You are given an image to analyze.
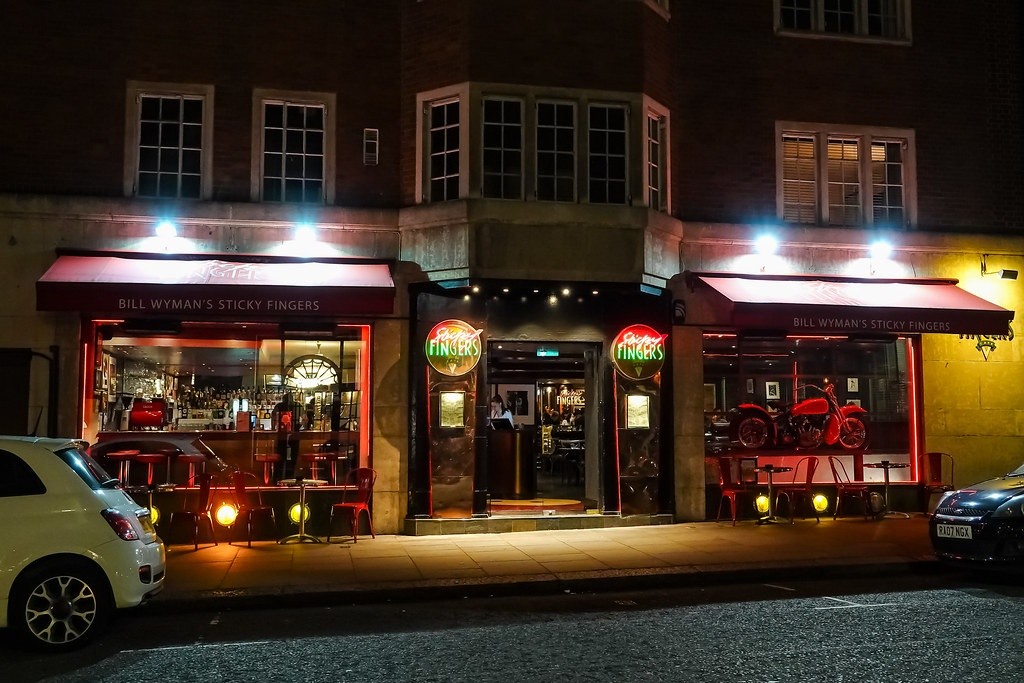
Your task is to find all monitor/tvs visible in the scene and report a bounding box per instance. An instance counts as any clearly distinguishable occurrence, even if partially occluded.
[490,418,513,430]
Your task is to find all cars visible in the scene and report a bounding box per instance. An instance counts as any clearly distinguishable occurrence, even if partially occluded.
[929,460,1024,574]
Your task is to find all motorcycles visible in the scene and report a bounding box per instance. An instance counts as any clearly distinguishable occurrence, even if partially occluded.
[728,377,874,451]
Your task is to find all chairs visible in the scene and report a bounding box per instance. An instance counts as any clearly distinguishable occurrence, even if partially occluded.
[166,473,221,550]
[714,456,763,526]
[917,453,955,515]
[828,456,874,520]
[775,457,820,525]
[225,471,278,548]
[327,468,378,544]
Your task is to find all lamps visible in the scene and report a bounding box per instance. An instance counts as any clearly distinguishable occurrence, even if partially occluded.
[980,255,1018,280]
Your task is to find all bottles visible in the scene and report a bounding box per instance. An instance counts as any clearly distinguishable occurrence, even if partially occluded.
[237,389,241,400]
[579,425,582,431]
[231,390,236,400]
[215,389,221,400]
[257,386,261,400]
[226,389,232,400]
[183,405,187,418]
[247,387,251,400]
[573,425,576,431]
[261,387,287,401]
[252,387,256,401]
[221,390,226,400]
[223,400,229,409]
[242,388,246,399]
[178,405,182,418]
[188,406,192,419]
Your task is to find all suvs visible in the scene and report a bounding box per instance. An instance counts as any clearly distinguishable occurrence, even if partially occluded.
[0,433,172,654]
[86,436,233,486]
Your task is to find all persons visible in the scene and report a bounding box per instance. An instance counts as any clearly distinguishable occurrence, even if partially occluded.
[542,406,585,426]
[271,393,310,484]
[491,394,514,428]
[306,398,326,429]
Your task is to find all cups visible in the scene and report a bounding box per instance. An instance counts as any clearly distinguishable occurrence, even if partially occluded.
[258,405,261,409]
[252,405,256,409]
[248,405,251,409]
[272,405,275,409]
[263,404,266,410]
[267,405,271,409]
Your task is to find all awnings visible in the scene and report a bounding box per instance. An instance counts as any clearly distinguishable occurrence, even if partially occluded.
[35,252,397,314]
[683,269,1015,338]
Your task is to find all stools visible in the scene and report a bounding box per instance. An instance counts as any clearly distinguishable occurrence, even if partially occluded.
[303,453,343,487]
[108,449,206,490]
[254,454,280,486]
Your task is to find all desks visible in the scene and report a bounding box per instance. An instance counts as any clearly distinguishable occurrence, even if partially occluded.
[128,484,174,517]
[861,461,911,518]
[746,464,793,524]
[278,478,328,544]
